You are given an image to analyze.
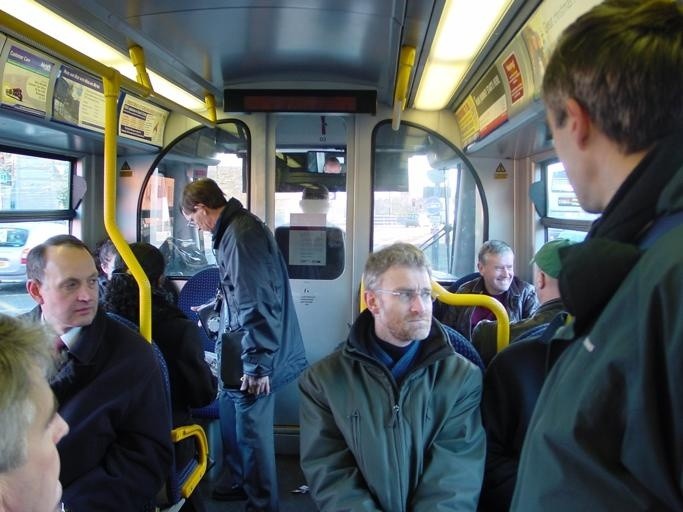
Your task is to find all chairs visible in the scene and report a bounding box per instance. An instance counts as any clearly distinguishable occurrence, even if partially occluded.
[430,312,494,511]
[175,267,251,481]
[98,311,209,511]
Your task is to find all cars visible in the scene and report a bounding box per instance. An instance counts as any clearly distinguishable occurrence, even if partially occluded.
[548,229,586,242]
[0,220,69,290]
[405,212,419,227]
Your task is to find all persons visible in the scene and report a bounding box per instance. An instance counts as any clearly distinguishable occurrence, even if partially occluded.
[295,240,489,512]
[177,177,311,512]
[507,1,683,510]
[471,238,579,360]
[299,181,330,219]
[0,313,72,512]
[9,233,175,512]
[442,238,539,344]
[97,241,219,472]
[89,236,128,305]
[323,157,341,174]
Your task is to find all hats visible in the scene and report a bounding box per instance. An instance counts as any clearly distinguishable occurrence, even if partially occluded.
[529,238,578,278]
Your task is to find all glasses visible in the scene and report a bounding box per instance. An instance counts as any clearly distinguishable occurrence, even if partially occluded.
[378,287,438,305]
[187,213,197,229]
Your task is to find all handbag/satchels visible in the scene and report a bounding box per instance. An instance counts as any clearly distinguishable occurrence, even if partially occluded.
[220,332,247,388]
